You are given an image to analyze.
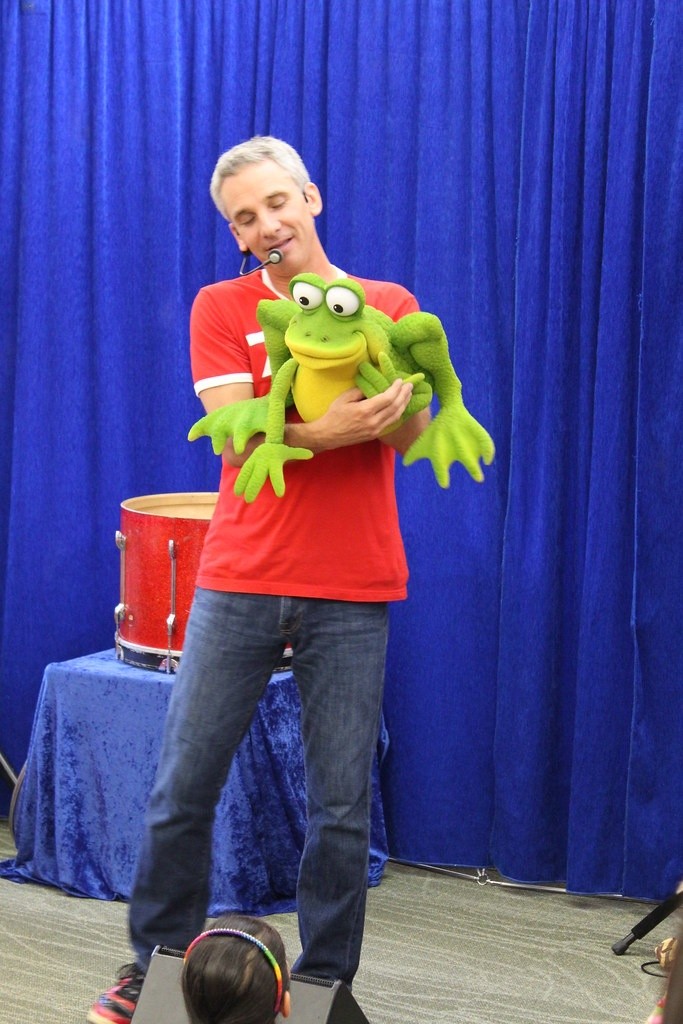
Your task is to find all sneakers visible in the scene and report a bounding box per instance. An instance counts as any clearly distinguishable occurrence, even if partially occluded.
[86,963,145,1024]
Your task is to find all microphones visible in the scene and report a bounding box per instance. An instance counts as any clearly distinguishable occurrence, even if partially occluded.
[239,248,284,275]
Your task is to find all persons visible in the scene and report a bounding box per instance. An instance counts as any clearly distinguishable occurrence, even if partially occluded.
[87,136,432,1024]
[180,914,291,1024]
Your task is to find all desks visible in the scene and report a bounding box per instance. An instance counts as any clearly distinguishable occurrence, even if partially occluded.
[0,648,390,918]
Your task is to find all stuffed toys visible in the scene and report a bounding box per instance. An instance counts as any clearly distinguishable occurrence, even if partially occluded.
[187,273,494,489]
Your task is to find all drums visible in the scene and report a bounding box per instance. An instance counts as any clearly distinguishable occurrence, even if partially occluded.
[111,487,297,676]
